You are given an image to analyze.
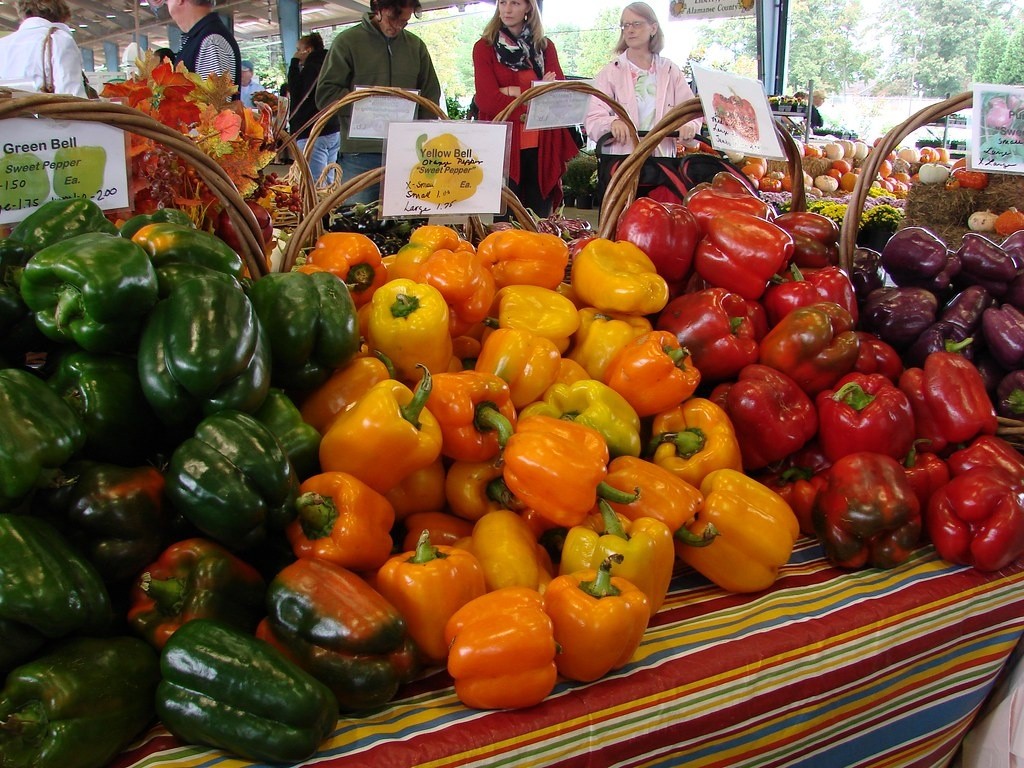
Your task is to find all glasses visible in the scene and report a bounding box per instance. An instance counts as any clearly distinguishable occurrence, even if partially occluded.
[619,20,648,30]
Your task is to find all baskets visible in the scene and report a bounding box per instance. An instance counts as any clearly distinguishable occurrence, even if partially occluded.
[0,81,1024,457]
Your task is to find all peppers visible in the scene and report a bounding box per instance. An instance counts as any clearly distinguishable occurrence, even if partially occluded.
[0,171,1024,768]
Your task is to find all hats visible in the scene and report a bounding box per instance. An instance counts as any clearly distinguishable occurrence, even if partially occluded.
[241,60,254,71]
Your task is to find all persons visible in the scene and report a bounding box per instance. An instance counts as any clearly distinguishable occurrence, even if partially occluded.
[794,92,810,100]
[315,0,441,211]
[584,1,702,200]
[155,48,181,65]
[471,0,580,223]
[287,31,340,187]
[166,0,241,102]
[0,0,90,100]
[241,60,266,108]
[277,82,290,148]
[800,89,828,129]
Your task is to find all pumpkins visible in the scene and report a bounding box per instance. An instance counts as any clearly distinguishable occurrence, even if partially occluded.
[684,137,1024,234]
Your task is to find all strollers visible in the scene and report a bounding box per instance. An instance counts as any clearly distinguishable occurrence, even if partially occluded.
[594,129,762,230]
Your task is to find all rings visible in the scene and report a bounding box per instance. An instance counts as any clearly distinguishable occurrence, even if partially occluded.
[689,132,693,136]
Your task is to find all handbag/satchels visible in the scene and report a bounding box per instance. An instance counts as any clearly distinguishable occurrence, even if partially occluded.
[40,27,99,100]
[568,125,584,146]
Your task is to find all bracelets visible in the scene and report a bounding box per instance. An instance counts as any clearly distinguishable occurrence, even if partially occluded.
[507,86,510,96]
